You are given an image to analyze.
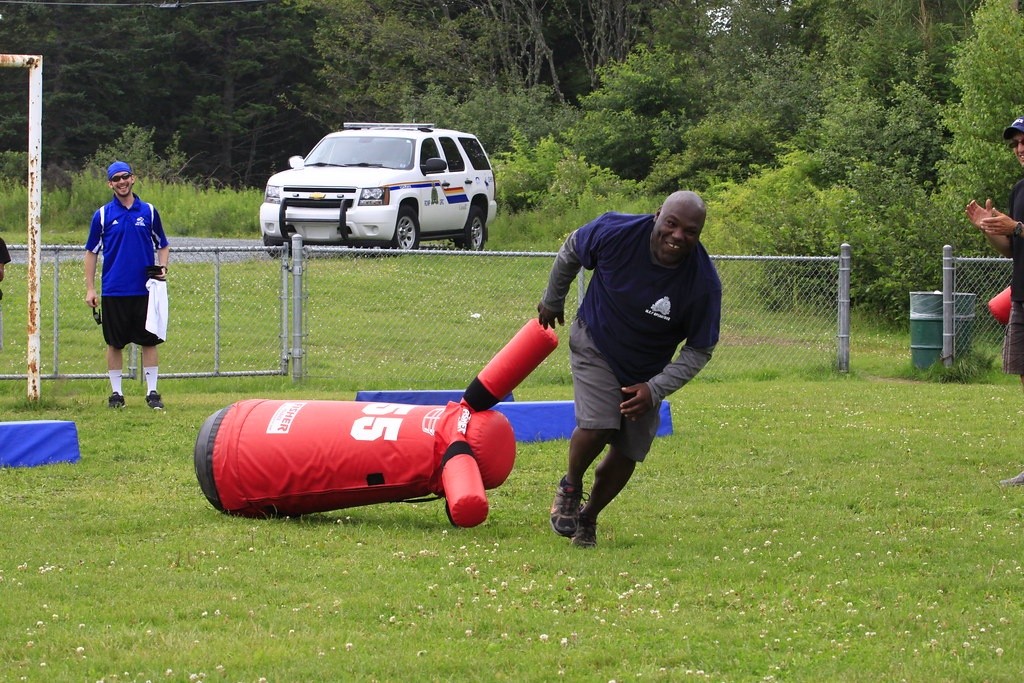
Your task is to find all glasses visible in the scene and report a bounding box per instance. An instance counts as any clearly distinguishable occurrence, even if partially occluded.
[1009,139,1024,148]
[110,173,132,182]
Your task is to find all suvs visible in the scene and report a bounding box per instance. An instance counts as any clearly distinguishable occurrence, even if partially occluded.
[258,121,498,260]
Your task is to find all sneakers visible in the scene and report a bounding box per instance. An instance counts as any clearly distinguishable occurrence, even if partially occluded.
[109,391,125,408]
[566,505,598,550]
[550,476,583,538]
[145,390,164,410]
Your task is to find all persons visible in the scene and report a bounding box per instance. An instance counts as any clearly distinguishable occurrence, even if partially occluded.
[534,191,724,549]
[83,160,171,409]
[965,117,1024,485]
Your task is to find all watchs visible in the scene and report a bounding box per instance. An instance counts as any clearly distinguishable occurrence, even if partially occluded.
[1014,220,1022,238]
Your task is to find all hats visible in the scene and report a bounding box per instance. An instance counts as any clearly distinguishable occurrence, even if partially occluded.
[1003,117,1024,139]
[108,162,132,180]
